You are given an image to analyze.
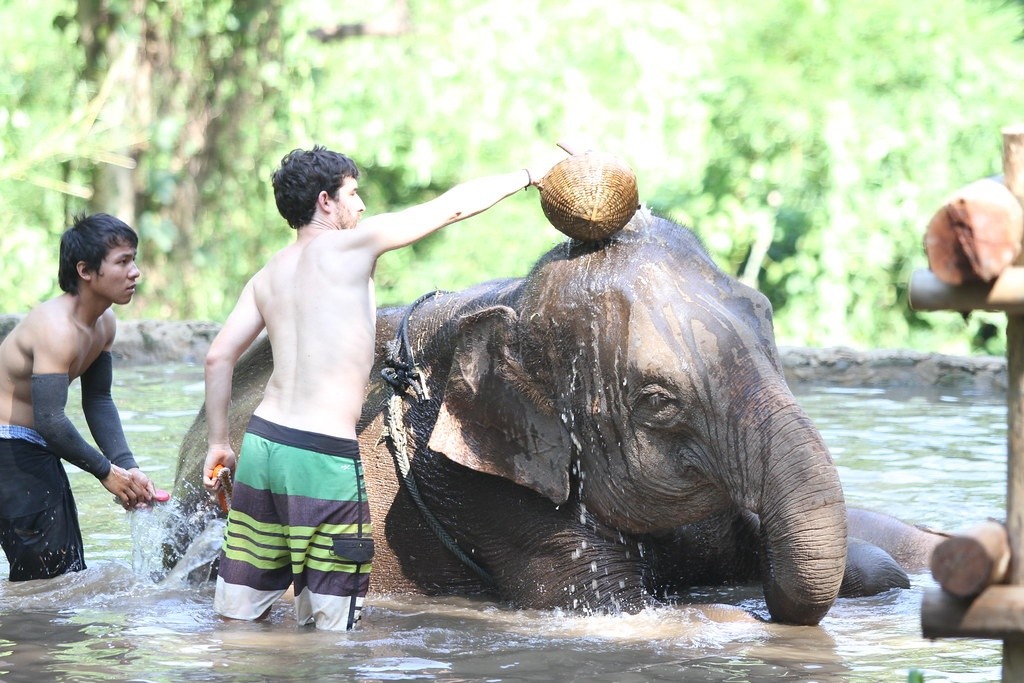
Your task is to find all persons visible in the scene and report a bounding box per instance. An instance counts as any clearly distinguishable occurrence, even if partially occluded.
[0,209,156,583]
[202,144,595,632]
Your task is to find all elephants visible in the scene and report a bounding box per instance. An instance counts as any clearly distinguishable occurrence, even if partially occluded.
[156,204,957,617]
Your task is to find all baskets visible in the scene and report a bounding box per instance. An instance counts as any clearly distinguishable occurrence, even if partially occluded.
[535,141,640,244]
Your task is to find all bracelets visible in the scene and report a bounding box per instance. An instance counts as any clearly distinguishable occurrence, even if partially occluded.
[522,169,531,191]
